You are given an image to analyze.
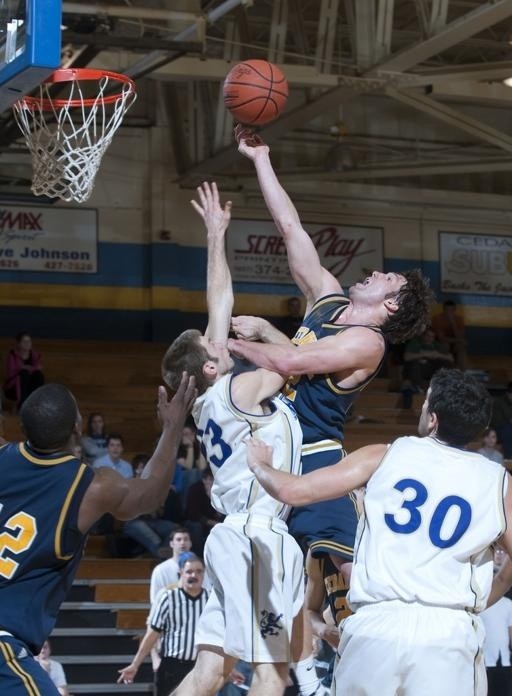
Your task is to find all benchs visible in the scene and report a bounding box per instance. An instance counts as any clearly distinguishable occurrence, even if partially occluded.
[468,350,511,395]
[48,530,161,696]
[0,333,170,466]
[345,358,434,457]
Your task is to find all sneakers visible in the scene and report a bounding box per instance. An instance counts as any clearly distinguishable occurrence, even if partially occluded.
[158,546,174,559]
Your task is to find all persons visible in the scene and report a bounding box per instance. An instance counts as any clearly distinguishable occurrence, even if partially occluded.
[164,178,307,696]
[225,120,433,696]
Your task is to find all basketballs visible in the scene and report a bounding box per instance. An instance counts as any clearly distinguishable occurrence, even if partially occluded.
[223,60,289,127]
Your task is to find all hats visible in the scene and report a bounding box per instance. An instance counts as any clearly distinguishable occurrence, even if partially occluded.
[179,551,196,568]
